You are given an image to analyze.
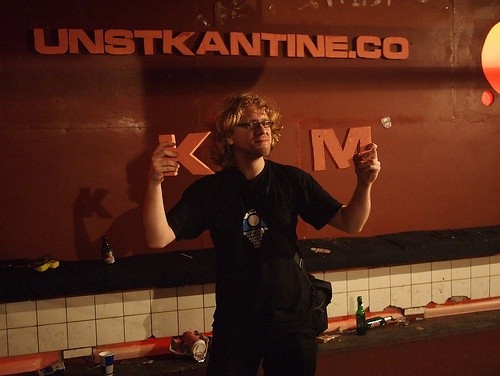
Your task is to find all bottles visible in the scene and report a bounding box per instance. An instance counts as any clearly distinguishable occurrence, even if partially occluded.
[366,316,393,329]
[101,236,115,267]
[355,296,366,335]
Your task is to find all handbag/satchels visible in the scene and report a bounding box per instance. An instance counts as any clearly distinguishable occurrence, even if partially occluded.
[308,272,333,335]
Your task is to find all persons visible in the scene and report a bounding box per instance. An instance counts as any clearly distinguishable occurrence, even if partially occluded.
[141,91,381,376]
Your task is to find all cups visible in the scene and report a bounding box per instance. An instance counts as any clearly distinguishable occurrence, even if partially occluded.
[98,351,114,376]
[182,331,206,355]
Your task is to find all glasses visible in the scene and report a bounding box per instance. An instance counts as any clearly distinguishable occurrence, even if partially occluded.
[238,121,273,130]
